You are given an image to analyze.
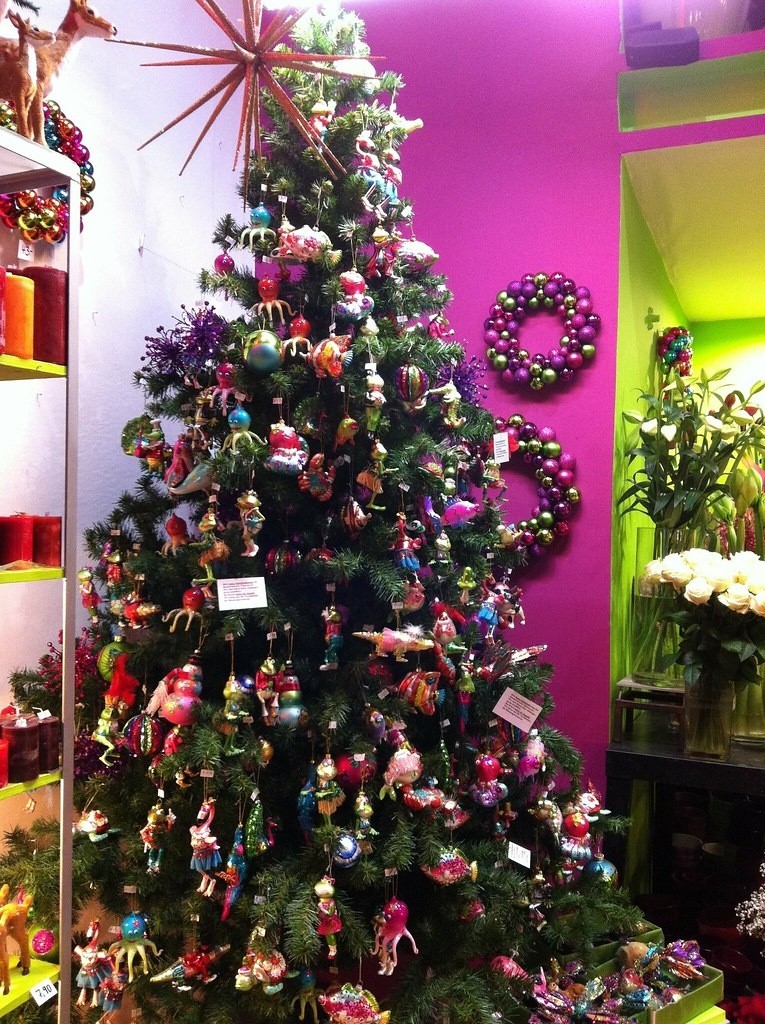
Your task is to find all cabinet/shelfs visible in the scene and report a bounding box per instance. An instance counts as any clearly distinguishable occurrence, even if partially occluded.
[0,128,87,1024]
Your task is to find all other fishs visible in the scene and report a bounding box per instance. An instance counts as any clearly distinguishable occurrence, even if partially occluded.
[379,748,424,802]
[317,984,391,1024]
[279,224,343,263]
[420,847,477,884]
[343,499,372,539]
[395,239,439,279]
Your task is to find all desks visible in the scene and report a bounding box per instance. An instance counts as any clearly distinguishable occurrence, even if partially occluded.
[602,711,765,892]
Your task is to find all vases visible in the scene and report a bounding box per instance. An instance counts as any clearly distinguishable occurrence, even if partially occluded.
[630,527,707,687]
[685,681,735,763]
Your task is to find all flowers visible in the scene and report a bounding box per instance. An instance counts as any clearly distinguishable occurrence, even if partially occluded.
[644,547,765,686]
[616,366,765,528]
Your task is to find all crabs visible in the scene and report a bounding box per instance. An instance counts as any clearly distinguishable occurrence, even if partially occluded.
[298,454,335,500]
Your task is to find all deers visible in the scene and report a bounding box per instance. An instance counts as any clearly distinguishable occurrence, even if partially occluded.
[0,0,117,147]
